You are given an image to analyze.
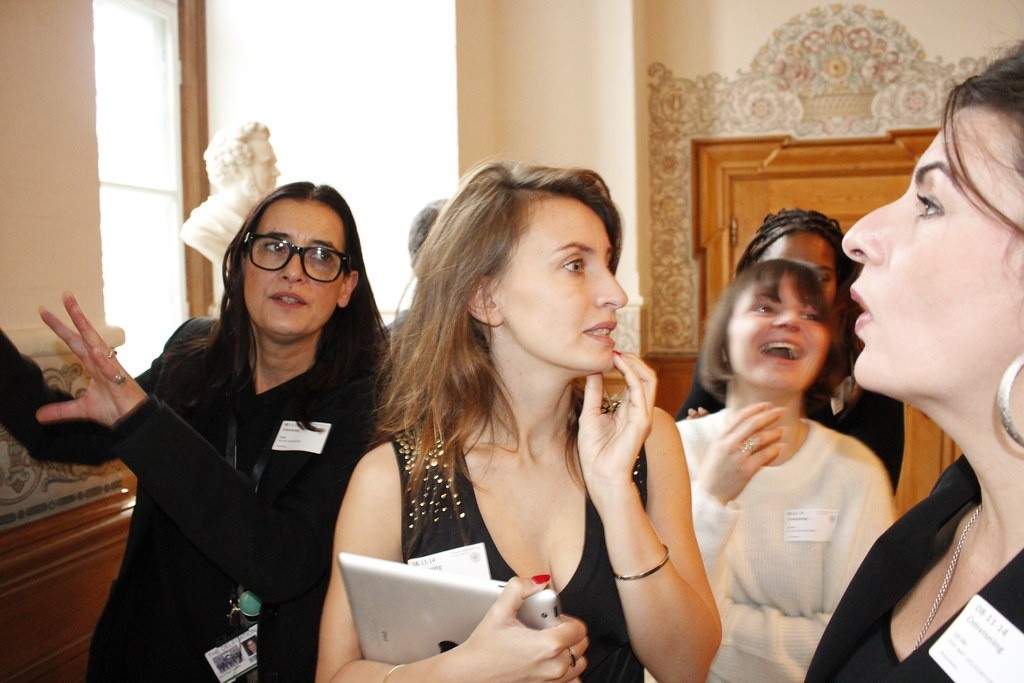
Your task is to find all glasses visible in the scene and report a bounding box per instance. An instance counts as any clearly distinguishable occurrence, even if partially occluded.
[244,232,353,283]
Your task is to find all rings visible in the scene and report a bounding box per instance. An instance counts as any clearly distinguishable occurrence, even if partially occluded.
[568,647,576,667]
[108,346,115,359]
[111,371,125,384]
[738,437,757,455]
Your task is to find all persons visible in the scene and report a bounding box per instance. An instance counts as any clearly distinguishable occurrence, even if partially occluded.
[806,42,1024,683]
[645,259,891,683]
[180,122,281,268]
[0,182,389,683]
[247,639,257,655]
[678,208,906,496]
[315,162,723,683]
[386,200,451,331]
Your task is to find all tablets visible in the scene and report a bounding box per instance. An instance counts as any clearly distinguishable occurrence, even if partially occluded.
[338,552,582,683]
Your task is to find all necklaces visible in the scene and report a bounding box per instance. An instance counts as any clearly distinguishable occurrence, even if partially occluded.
[914,504,983,651]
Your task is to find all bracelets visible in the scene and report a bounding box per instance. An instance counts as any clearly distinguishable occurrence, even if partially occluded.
[382,664,406,683]
[615,544,670,581]
